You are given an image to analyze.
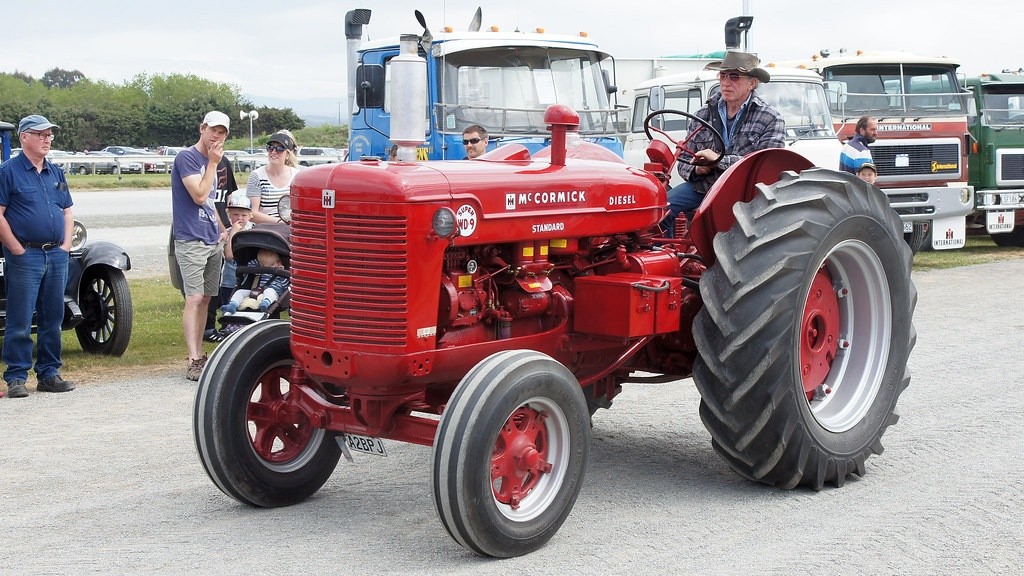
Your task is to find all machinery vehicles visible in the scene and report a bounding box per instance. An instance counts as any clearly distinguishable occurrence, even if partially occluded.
[191,100,915,560]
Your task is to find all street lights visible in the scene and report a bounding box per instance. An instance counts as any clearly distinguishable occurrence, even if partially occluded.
[240,109,259,172]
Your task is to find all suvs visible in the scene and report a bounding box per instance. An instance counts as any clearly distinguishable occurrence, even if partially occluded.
[298,147,341,167]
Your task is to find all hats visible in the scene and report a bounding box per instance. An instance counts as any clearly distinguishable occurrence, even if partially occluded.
[266,134,297,152]
[202,111,230,135]
[858,162,876,174]
[18,115,60,137]
[227,191,251,210]
[706,51,770,83]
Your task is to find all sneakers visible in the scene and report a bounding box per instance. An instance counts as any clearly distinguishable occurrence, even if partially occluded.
[199,352,207,366]
[203,329,224,342]
[186,359,202,380]
[8,379,29,397]
[37,375,75,391]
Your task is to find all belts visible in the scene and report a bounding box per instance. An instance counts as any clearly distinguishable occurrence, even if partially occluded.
[22,241,63,250]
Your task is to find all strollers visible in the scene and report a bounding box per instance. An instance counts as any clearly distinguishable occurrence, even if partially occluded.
[219,220,292,337]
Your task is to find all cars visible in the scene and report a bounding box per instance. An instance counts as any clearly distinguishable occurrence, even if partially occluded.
[10,145,187,175]
[223,149,269,172]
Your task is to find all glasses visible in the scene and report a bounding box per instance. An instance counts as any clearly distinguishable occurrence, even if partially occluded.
[265,143,288,154]
[716,72,753,82]
[462,138,483,145]
[25,132,54,140]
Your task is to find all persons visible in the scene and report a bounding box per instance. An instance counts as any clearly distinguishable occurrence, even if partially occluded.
[859,162,877,185]
[219,250,289,314]
[217,191,256,306]
[0,113,76,398]
[203,155,239,341]
[838,115,878,176]
[462,125,490,160]
[660,50,785,239]
[246,129,302,227]
[171,110,230,383]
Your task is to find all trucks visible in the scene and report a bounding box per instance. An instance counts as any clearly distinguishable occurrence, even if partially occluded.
[313,6,1024,259]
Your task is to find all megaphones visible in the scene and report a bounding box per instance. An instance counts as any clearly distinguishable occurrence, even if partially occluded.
[249,110,259,120]
[240,110,250,120]
